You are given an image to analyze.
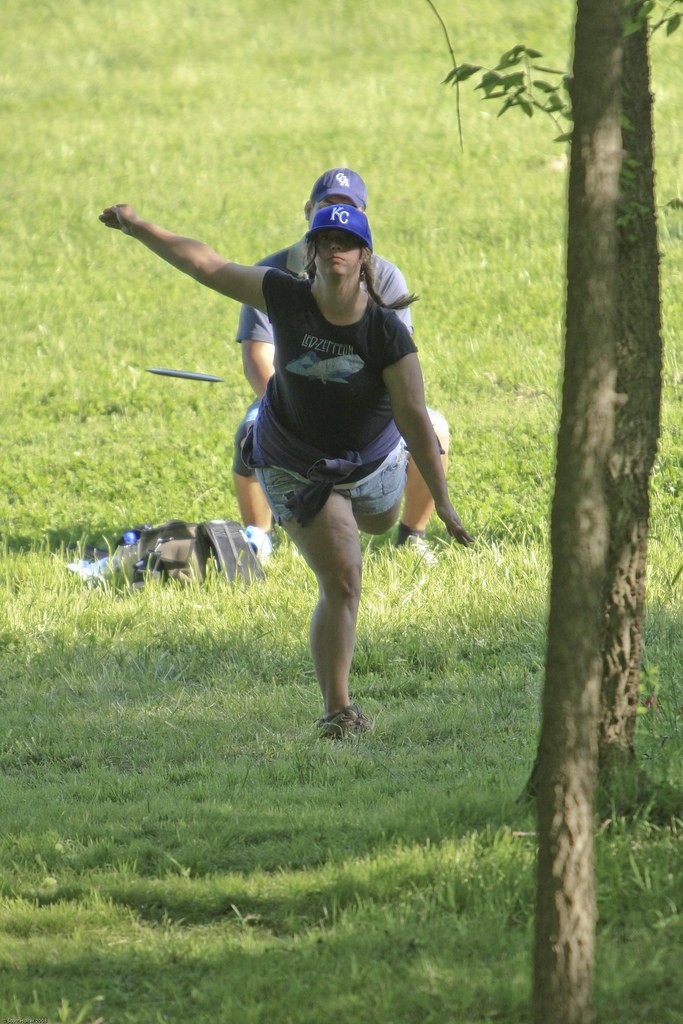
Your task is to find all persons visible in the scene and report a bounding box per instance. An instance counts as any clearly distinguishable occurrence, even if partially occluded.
[98,204,474,740]
[231,169,448,568]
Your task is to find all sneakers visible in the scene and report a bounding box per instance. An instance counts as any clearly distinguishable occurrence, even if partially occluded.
[317,704,373,741]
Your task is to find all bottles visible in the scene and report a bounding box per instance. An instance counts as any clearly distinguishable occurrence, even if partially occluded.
[120,533,138,566]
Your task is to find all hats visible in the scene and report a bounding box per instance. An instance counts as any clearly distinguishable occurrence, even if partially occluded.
[305,204,372,252]
[312,169,365,212]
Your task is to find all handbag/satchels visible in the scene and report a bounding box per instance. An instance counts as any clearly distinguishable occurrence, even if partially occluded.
[131,519,266,586]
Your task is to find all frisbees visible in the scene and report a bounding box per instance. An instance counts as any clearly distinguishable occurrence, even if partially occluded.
[145,368,224,383]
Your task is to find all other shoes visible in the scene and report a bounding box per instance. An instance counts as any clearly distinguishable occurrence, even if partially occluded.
[394,538,434,565]
